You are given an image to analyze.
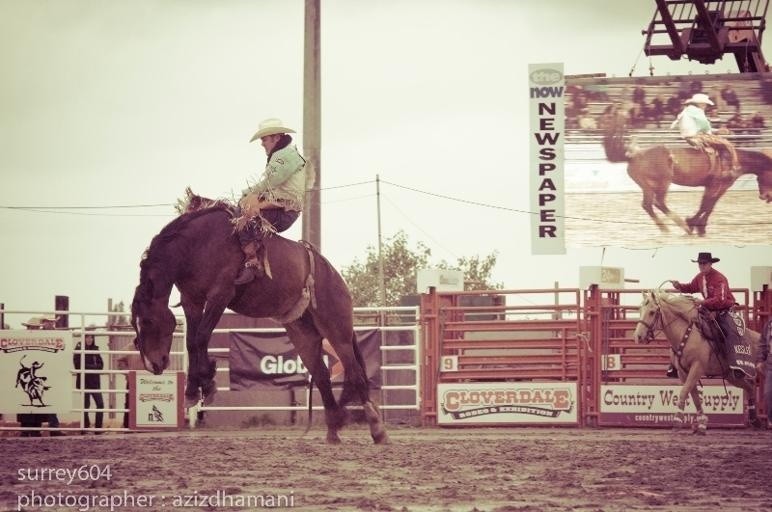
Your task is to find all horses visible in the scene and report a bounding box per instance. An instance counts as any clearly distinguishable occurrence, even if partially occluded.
[127,205,388,445]
[599,110,772,238]
[634,288,772,433]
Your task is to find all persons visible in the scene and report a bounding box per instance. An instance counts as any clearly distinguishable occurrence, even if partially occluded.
[19,316,45,437]
[40,313,65,435]
[669,251,747,382]
[229,117,310,281]
[755,319,772,425]
[117,332,146,432]
[73,334,104,433]
[563,84,771,129]
[668,92,733,178]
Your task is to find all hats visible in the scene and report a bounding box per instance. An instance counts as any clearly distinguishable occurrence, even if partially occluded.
[680,94,714,107]
[40,314,61,323]
[21,317,46,327]
[249,118,296,142]
[689,252,720,263]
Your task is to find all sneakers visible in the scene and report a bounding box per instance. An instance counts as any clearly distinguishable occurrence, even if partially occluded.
[19,428,104,437]
[666,366,677,377]
[732,366,745,379]
[233,261,267,285]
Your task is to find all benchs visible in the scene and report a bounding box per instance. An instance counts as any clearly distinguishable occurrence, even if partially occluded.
[563,77,772,149]
